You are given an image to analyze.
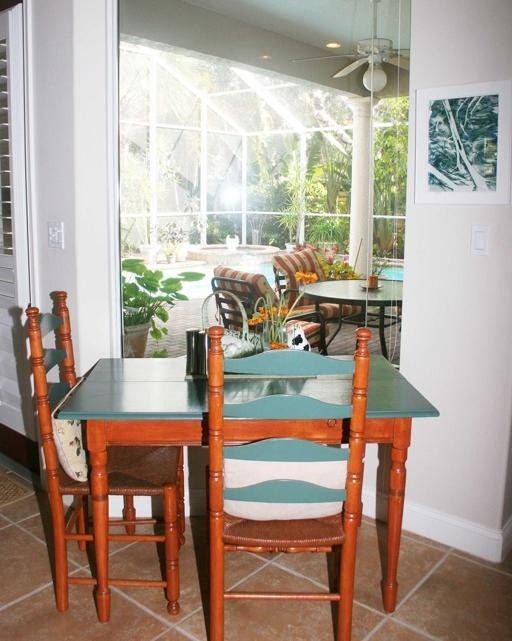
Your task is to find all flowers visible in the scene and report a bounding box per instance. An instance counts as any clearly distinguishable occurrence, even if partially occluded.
[246,269,317,350]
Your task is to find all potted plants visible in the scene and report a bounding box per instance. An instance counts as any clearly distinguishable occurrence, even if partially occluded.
[121,255,205,356]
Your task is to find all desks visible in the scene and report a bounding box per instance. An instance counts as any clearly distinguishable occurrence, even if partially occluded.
[51,355,436,620]
[297,274,405,364]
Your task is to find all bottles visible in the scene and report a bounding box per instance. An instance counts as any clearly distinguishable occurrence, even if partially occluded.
[186,328,209,376]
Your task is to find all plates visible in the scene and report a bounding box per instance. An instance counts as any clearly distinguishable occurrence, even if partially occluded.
[359,283,384,289]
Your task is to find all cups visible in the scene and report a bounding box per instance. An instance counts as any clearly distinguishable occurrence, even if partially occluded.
[367,274,378,288]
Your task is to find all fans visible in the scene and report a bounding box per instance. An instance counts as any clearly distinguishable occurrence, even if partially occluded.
[290,0,410,78]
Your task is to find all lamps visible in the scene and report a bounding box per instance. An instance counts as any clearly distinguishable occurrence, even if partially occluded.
[363,0,388,93]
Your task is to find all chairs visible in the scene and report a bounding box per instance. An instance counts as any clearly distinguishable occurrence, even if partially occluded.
[194,321,373,641]
[268,248,359,332]
[26,288,186,619]
[209,266,331,355]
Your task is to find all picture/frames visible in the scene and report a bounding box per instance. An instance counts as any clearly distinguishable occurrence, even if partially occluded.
[415,80,510,204]
